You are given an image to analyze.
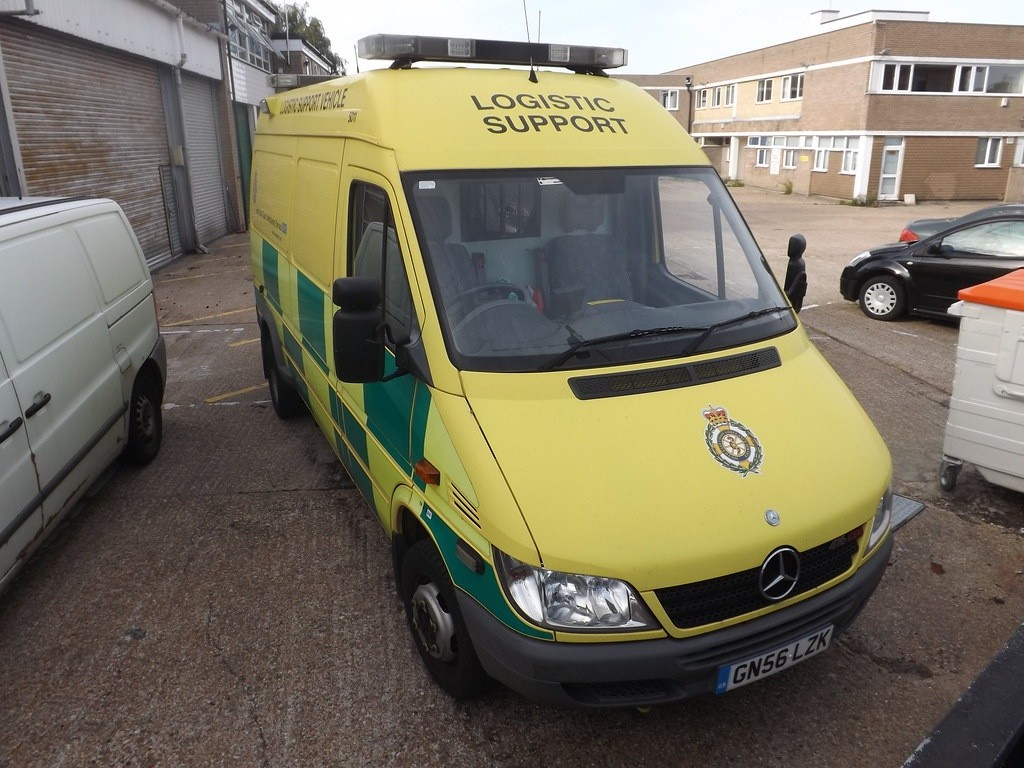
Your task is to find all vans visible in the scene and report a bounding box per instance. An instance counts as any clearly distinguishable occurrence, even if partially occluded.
[0,195,168,590]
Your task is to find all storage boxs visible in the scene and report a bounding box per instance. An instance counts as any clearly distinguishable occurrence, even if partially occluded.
[941,268,1024,493]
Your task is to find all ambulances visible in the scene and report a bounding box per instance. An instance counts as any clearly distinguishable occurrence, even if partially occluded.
[249,35,897,707]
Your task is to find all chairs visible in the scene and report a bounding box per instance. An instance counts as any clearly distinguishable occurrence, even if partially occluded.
[414,193,487,315]
[535,190,639,312]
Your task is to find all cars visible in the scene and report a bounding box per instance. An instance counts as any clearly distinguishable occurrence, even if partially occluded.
[900,216,1023,255]
[839,203,1024,323]
